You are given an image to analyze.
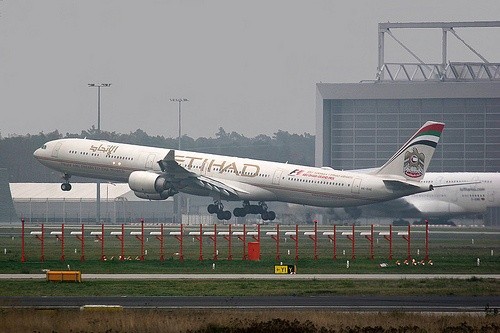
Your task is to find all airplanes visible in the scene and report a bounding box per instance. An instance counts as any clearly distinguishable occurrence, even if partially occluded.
[288,165,499,223]
[32,118,448,222]
